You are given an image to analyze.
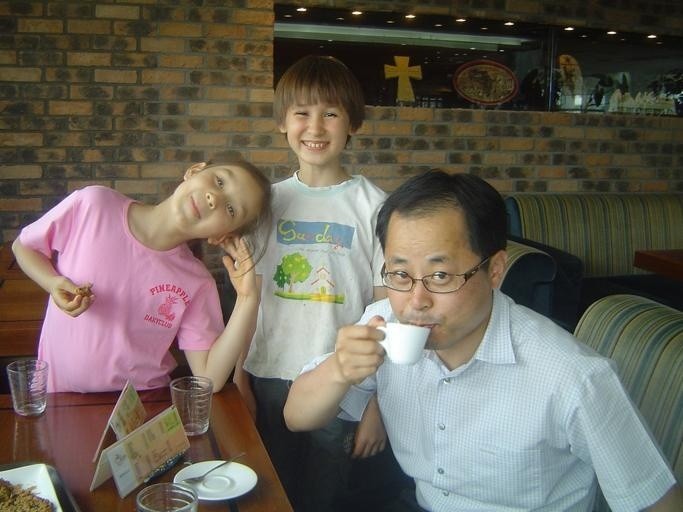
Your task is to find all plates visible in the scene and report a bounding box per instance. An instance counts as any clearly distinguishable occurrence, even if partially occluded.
[0,463,65,512]
[172,460,258,502]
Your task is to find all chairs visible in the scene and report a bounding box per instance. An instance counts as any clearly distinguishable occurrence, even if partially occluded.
[574,289,681,471]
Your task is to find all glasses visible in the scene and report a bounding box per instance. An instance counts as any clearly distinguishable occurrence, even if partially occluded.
[381,258,492,295]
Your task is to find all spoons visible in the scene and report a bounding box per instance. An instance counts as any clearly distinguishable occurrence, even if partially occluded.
[179,453,250,484]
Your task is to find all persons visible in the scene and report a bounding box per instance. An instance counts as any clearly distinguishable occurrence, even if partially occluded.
[231,55,393,511]
[11,156,274,396]
[281,168,682,511]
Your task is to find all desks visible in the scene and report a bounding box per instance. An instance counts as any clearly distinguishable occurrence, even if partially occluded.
[635,245,683,276]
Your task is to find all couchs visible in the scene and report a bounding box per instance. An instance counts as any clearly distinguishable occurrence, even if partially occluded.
[495,190,683,320]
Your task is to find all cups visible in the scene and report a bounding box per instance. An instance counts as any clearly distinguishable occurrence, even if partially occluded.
[375,321,431,366]
[135,482,198,512]
[7,359,49,417]
[170,376,212,435]
